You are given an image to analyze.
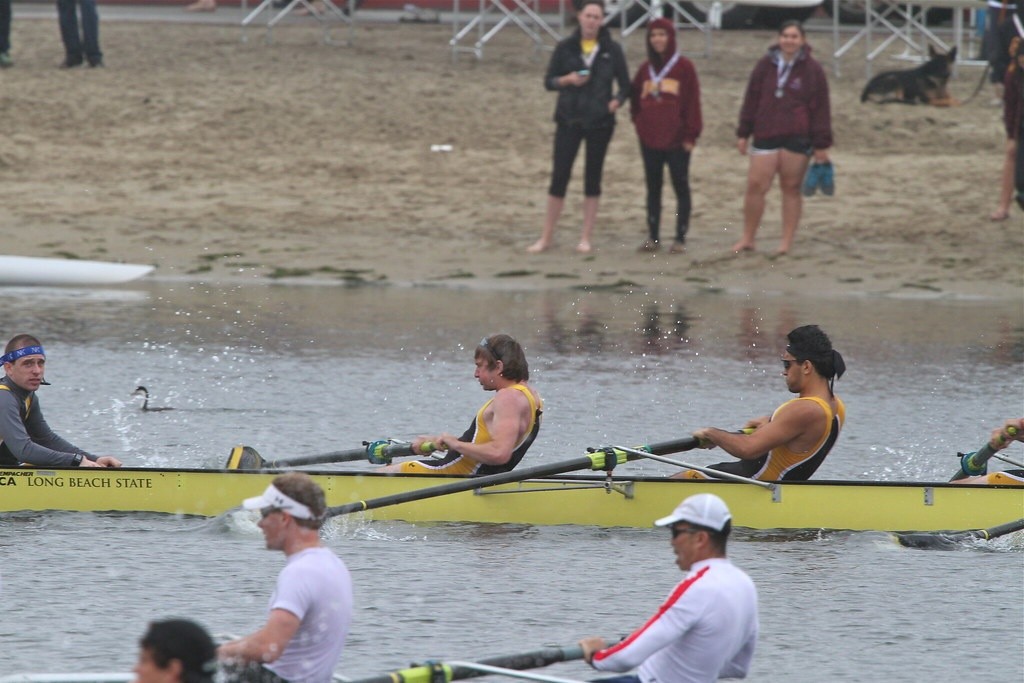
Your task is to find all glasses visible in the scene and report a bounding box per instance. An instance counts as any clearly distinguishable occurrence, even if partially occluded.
[480,337,500,359]
[671,527,694,538]
[783,359,798,370]
[260,508,281,518]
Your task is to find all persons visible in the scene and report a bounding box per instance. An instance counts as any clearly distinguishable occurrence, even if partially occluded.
[731,21,832,255]
[132,619,215,683]
[981,0,1024,221]
[377,335,543,474]
[579,494,758,683]
[0,334,123,466]
[0,0,14,66]
[54,0,101,68]
[669,325,845,480]
[527,0,630,253]
[950,417,1024,485]
[630,19,702,253]
[216,475,353,683]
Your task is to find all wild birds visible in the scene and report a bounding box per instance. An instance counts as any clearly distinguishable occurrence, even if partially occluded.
[129,385,175,411]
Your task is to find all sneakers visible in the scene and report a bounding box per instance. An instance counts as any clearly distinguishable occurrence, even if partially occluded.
[820,161,834,196]
[802,162,820,196]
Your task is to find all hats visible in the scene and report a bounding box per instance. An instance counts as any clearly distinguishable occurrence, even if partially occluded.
[242,483,311,519]
[654,493,731,536]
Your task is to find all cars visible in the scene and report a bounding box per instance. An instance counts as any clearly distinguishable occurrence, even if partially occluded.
[571,0,825,31]
[823,0,986,26]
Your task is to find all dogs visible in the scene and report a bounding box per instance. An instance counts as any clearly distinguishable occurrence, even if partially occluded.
[860,44,958,106]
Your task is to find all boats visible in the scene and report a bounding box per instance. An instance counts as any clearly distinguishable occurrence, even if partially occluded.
[0,256,157,287]
[0,461,1024,530]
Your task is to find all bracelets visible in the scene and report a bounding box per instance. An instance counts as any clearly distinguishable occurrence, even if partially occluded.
[590,650,596,663]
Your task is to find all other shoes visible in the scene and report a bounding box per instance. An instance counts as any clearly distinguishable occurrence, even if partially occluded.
[0,52,13,65]
[61,53,84,67]
[670,244,686,255]
[641,238,661,252]
[86,53,102,68]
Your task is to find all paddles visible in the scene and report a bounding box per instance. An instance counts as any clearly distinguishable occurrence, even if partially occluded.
[344,639,621,683]
[326,427,757,516]
[227,441,446,469]
[948,426,1016,482]
[979,519,1024,540]
